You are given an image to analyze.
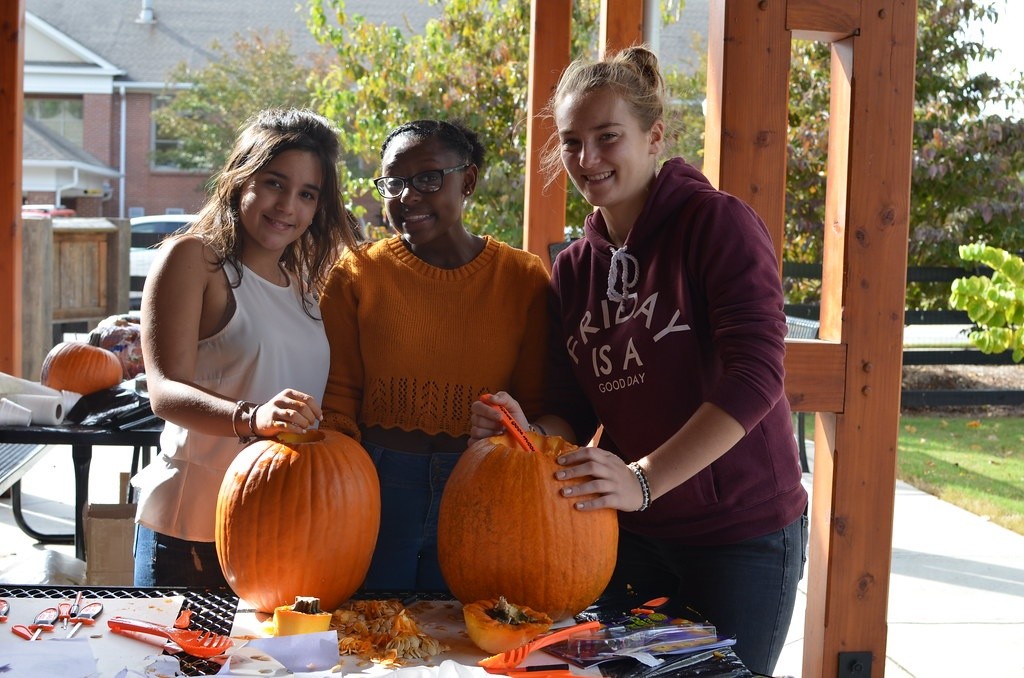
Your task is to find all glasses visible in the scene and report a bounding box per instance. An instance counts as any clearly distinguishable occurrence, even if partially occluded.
[373,163,469,199]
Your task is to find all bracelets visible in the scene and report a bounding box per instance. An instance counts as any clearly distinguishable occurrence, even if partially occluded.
[249,402,262,438]
[628,462,651,512]
[231,399,254,445]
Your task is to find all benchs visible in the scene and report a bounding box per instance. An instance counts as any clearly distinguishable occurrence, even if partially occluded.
[0,441,53,540]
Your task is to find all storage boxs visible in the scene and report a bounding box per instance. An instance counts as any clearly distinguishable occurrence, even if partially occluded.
[82,502,136,586]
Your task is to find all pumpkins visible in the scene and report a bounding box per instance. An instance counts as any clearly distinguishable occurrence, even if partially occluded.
[40,313,143,396]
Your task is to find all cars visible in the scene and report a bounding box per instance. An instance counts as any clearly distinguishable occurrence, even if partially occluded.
[128,212,202,310]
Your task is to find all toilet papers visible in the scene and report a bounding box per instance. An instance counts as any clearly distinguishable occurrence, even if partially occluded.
[0,394,65,425]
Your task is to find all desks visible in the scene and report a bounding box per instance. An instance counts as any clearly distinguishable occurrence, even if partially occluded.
[0,596,747,678]
[0,398,165,561]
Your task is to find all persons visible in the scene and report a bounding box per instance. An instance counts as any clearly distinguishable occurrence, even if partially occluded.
[310,118,561,607]
[135,108,357,591]
[467,44,810,676]
